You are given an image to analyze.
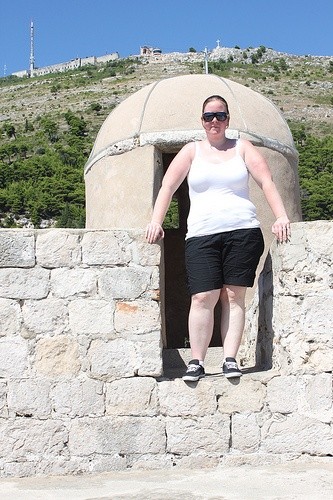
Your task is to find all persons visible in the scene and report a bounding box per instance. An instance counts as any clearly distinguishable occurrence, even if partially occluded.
[145,94,291,382]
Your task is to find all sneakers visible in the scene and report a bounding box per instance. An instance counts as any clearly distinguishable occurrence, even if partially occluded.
[182,359,205,381]
[222,357,242,378]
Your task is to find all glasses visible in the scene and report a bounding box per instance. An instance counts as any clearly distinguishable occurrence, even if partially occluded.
[202,112,228,122]
